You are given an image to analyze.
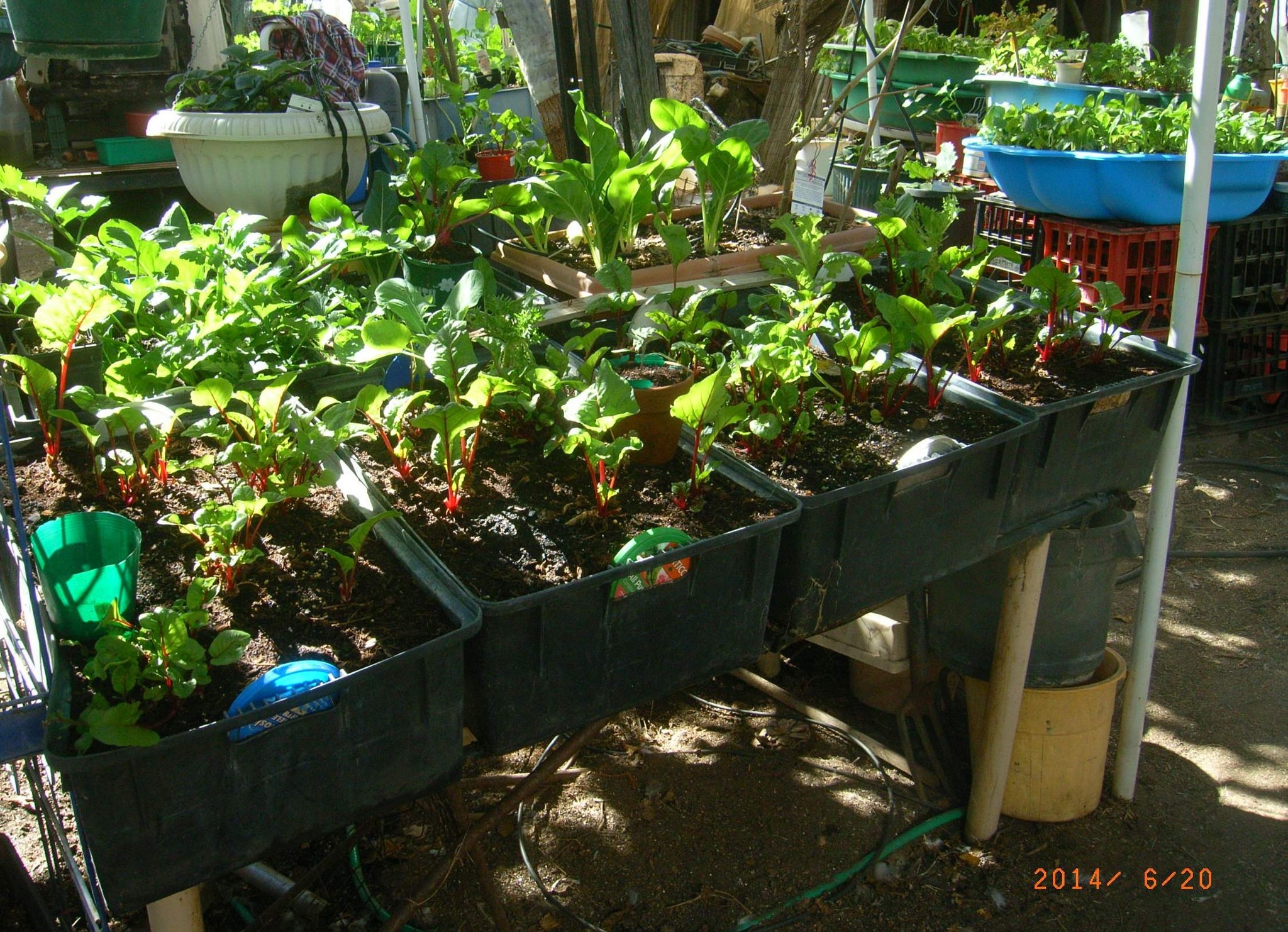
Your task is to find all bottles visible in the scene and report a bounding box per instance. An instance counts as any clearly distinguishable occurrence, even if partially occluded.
[896,435,968,494]
[608,528,702,601]
[1221,74,1252,111]
[359,60,403,145]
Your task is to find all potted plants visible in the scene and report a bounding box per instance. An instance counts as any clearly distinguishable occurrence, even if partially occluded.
[0,0,1288,914]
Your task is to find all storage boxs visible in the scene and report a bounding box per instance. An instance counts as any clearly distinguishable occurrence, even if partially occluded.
[1196,323,1288,436]
[969,195,1070,295]
[1040,214,1218,341]
[1204,208,1288,337]
[93,135,177,166]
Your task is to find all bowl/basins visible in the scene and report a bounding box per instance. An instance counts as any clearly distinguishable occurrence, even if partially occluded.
[125,113,154,137]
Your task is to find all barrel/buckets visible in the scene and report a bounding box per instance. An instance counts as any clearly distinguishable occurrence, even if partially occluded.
[925,510,1136,686]
[960,645,1128,821]
[345,158,368,203]
[962,147,989,177]
[225,660,341,741]
[30,511,142,641]
[383,348,431,392]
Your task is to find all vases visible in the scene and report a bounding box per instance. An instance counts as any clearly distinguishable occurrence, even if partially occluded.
[852,660,916,716]
[960,643,1129,826]
[926,504,1133,686]
